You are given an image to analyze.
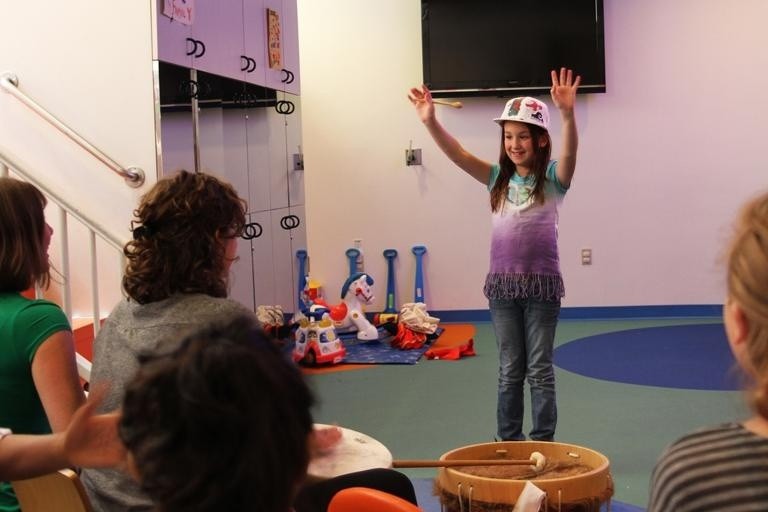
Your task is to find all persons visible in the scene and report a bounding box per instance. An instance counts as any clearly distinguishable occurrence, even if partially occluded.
[647,192,768,511]
[407,67,581,442]
[0,382,128,512]
[75,171,341,511]
[118,313,317,512]
[0,178,86,436]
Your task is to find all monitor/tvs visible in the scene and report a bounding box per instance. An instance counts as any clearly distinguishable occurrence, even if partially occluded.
[421,0,606,97]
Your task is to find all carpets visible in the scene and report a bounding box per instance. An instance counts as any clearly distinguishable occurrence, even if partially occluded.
[282,326,446,364]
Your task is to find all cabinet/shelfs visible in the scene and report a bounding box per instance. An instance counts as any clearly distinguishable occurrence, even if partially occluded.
[151,1,308,329]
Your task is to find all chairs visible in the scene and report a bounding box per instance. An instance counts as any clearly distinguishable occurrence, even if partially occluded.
[12,464,92,510]
[326,485,423,510]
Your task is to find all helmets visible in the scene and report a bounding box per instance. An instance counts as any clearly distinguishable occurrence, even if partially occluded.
[493,96,549,131]
